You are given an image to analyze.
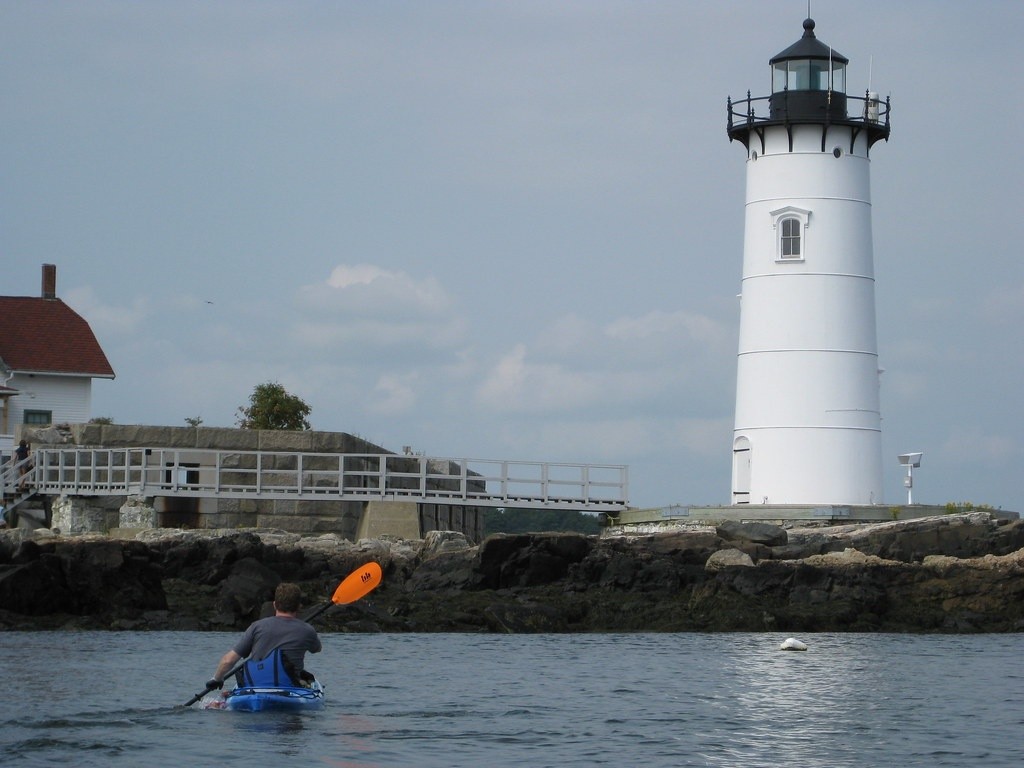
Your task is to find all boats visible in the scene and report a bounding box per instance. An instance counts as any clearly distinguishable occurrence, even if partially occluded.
[223,648,326,713]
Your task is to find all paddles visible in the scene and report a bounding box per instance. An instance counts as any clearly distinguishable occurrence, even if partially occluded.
[184,562,382,707]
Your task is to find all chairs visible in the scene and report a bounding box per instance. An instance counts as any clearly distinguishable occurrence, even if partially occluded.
[240,648,293,687]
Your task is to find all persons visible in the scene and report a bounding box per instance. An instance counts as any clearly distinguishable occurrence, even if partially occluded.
[0,499,7,529]
[13,439,30,488]
[210,583,322,687]
